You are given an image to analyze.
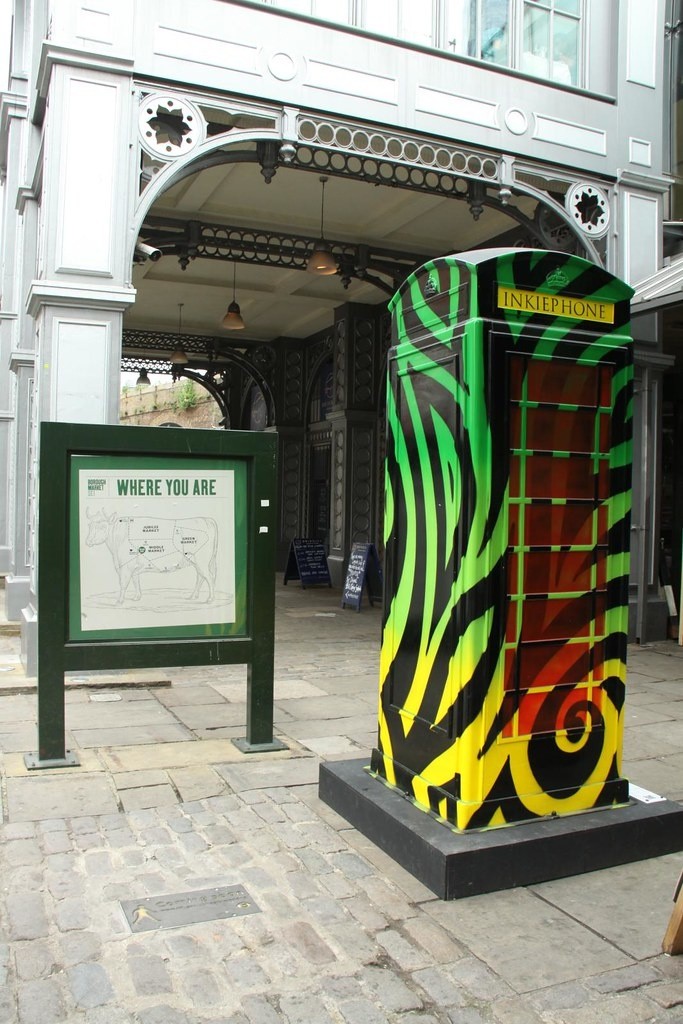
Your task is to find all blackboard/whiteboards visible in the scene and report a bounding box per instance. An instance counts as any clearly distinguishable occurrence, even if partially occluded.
[292,538,332,585]
[342,541,372,606]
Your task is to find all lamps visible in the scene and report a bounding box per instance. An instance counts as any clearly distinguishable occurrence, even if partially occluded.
[170,304,188,363]
[306,177,338,276]
[137,349,150,386]
[223,255,245,329]
